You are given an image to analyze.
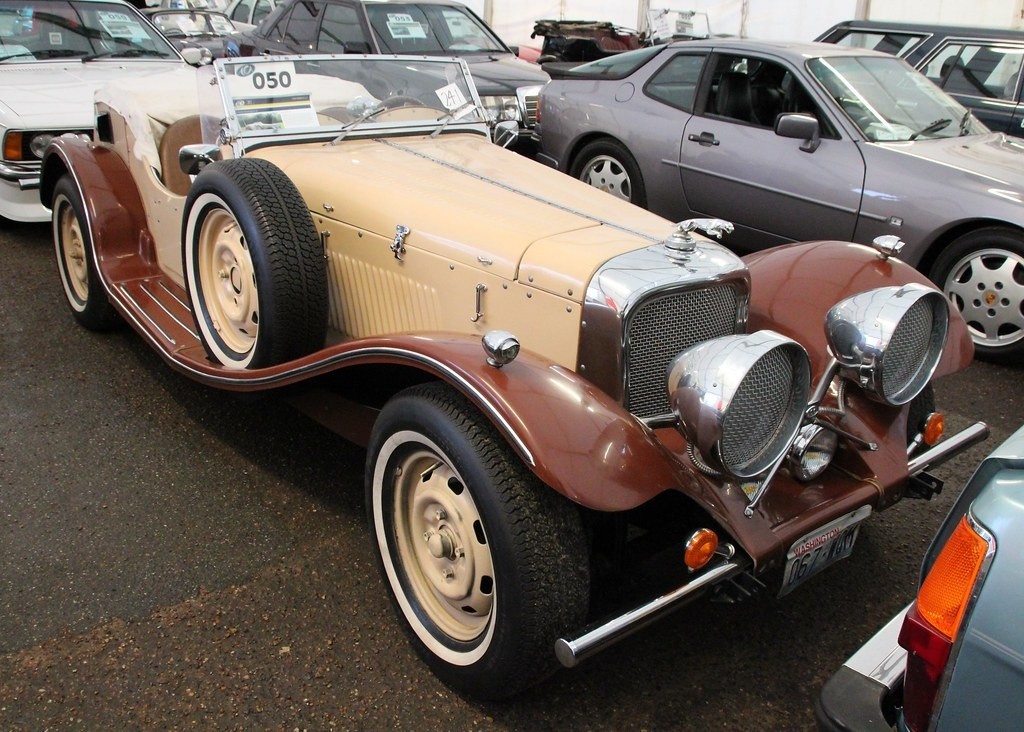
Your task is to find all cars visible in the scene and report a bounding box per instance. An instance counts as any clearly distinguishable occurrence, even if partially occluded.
[533,40,1024,363]
[0,0,197,223]
[815,424,1024,732]
[224,0,552,158]
[221,0,289,58]
[140,8,237,63]
[40,47,995,712]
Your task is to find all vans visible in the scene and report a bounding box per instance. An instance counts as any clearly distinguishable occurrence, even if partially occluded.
[812,20,1024,145]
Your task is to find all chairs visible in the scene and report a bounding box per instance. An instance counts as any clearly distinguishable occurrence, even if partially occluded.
[318,106,360,126]
[159,114,222,196]
[715,72,762,127]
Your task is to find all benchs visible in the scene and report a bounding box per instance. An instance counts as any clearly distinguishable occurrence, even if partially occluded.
[709,84,782,128]
